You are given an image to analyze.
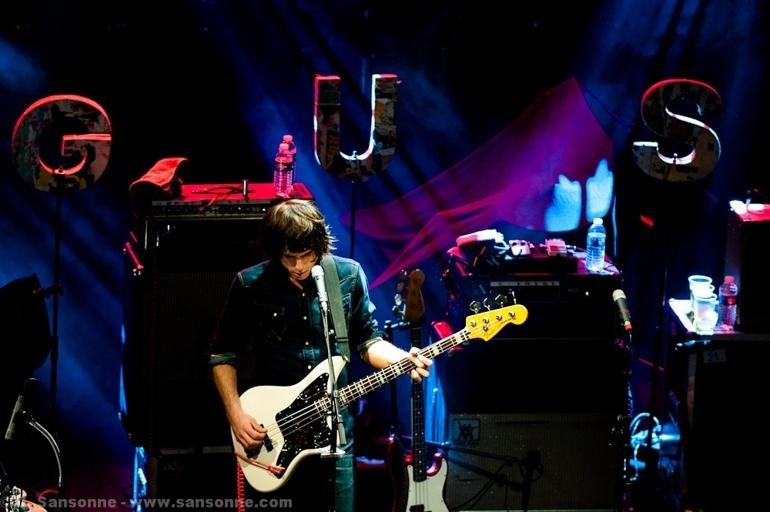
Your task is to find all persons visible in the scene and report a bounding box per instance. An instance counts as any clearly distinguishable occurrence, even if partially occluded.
[206,198,435,511]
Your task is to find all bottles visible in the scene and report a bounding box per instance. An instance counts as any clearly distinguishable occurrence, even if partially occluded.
[274,134,297,195]
[718,277,739,330]
[586,218,608,276]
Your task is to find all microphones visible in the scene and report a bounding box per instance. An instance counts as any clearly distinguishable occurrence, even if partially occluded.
[311,265,328,316]
[612,288,633,331]
[4,381,29,440]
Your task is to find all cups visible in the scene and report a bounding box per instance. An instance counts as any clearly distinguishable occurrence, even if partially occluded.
[689,273,718,334]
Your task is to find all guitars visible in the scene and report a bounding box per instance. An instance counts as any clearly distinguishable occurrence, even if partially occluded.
[368,268,451,512]
[231,287,529,492]
